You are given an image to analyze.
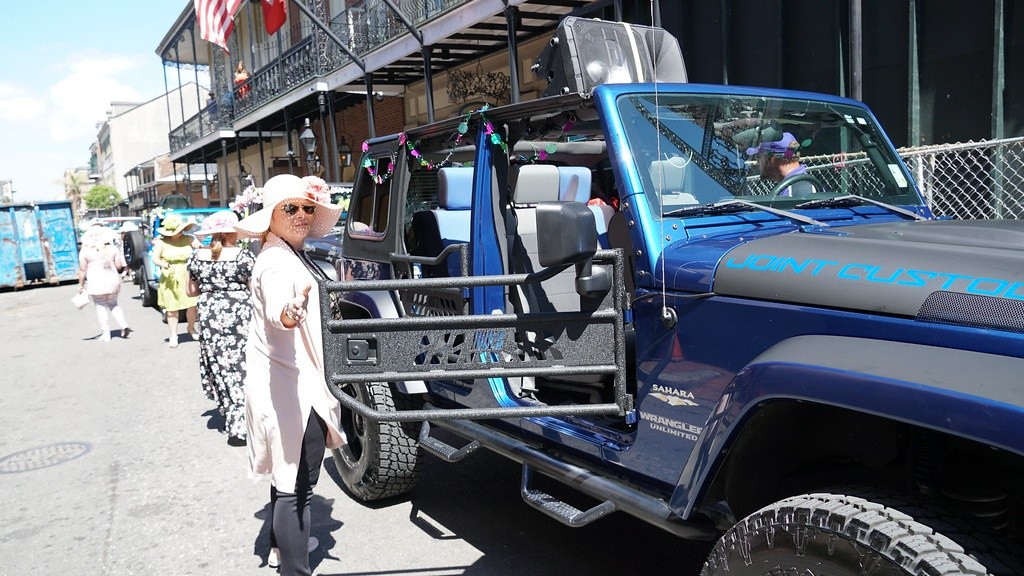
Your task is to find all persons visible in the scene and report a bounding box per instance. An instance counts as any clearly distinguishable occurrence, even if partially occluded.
[186,211,257,447]
[222,85,234,120]
[746,132,817,196]
[235,62,249,94]
[207,91,217,121]
[152,216,199,348]
[80,218,138,343]
[235,174,344,576]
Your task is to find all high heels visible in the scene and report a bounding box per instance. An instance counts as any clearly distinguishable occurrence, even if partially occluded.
[187,326,199,340]
[169,335,178,347]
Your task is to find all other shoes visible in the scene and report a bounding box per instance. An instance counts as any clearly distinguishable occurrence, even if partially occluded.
[124,276,133,281]
[228,433,246,446]
[120,271,127,276]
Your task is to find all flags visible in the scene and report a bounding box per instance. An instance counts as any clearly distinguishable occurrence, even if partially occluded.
[193,0,288,56]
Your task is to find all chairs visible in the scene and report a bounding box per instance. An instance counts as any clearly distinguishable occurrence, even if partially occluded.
[412,161,595,312]
[649,158,699,206]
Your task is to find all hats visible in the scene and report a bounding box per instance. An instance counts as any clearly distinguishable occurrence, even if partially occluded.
[91,218,104,226]
[746,133,797,155]
[80,225,117,247]
[117,221,140,233]
[156,213,199,236]
[232,174,343,238]
[193,210,238,242]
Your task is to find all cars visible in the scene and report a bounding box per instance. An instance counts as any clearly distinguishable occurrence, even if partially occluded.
[92,214,142,229]
[249,181,352,283]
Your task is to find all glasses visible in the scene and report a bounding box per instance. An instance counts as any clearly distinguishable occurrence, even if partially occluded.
[274,204,317,215]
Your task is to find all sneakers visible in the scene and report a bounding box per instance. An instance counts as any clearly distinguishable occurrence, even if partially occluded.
[267,536,319,566]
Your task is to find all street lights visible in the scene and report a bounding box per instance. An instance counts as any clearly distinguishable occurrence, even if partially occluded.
[299,117,321,177]
[88,174,101,218]
[9,180,15,203]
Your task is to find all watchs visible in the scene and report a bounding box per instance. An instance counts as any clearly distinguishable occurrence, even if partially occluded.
[284,303,289,316]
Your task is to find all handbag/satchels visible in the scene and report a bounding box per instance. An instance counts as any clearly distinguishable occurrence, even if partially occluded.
[186,271,201,296]
[71,289,91,308]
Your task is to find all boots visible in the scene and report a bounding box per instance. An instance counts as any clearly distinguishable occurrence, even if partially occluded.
[95,304,111,342]
[112,305,130,337]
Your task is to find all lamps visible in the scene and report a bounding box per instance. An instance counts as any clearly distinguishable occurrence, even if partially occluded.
[338,97,353,167]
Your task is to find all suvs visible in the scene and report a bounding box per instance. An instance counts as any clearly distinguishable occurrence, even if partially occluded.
[123,192,243,307]
[253,15,1024,575]
